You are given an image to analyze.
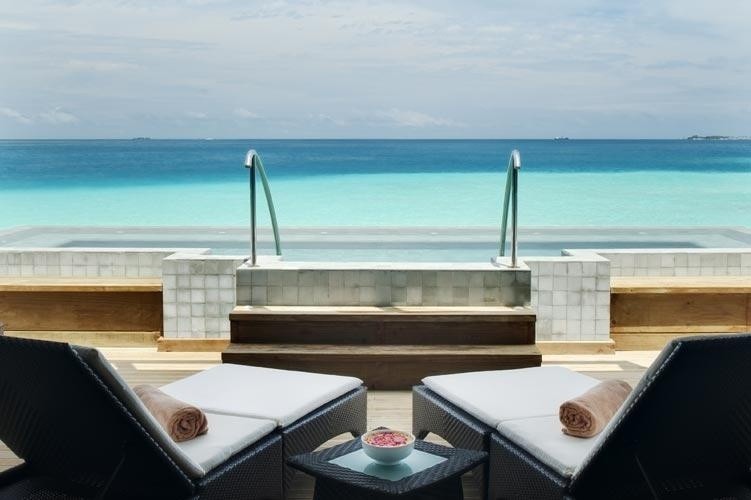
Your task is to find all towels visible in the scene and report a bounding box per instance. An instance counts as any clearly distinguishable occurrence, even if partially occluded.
[559,378,633,439]
[129,384,209,443]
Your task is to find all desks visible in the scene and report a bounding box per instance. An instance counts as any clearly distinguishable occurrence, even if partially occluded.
[288,425,491,500]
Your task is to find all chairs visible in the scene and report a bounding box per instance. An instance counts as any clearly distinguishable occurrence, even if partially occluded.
[0,335,368,498]
[414,335,751,499]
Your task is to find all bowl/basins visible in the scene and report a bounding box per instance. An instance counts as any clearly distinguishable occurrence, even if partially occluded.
[361,429,416,466]
